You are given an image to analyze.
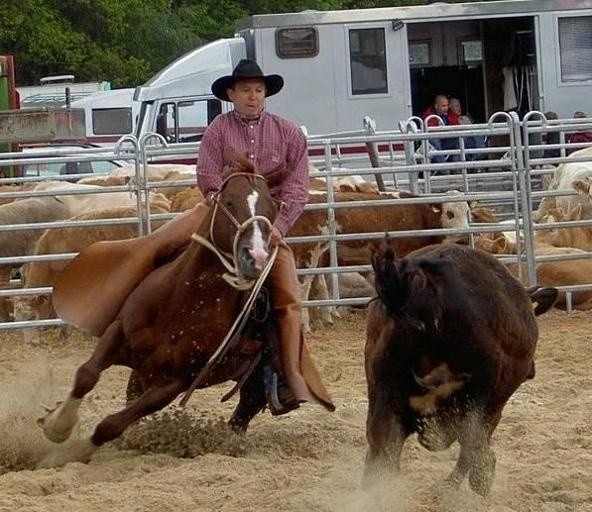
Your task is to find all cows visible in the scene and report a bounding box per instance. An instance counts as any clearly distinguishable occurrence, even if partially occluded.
[0,147,592,347]
[359,229,560,496]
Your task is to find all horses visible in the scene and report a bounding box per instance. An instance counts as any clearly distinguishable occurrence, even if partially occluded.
[35,142,293,469]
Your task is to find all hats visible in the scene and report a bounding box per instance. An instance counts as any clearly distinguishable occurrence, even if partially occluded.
[210,58,284,102]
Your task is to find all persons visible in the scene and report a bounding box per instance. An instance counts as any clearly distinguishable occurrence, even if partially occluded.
[447,96,487,174]
[565,110,592,156]
[53,59,337,420]
[538,110,569,169]
[416,94,469,177]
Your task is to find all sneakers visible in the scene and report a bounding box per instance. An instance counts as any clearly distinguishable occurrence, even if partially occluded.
[275,379,294,405]
